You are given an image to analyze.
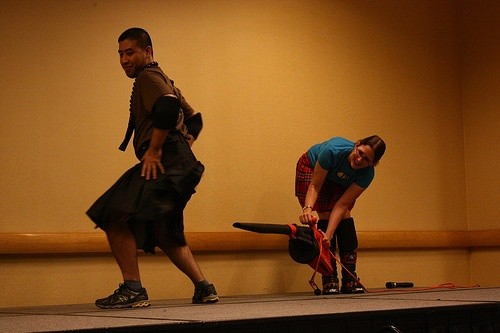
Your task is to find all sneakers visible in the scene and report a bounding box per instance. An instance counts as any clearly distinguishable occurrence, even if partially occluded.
[95,283,152,309]
[192,284,220,304]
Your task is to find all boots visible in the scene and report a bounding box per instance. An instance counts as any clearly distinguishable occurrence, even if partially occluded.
[317,219,340,294]
[335,217,365,294]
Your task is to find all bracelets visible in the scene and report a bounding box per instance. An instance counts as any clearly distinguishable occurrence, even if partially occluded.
[302,205,313,211]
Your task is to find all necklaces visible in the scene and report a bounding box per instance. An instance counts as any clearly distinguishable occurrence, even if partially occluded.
[129,61,159,111]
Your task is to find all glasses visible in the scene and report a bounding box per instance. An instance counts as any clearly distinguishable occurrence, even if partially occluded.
[355,144,376,166]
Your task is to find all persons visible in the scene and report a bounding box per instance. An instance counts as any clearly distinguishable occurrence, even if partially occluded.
[85,26,220,308]
[295,135,386,293]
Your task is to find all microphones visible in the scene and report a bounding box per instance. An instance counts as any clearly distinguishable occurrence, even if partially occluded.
[385,282,414,289]
